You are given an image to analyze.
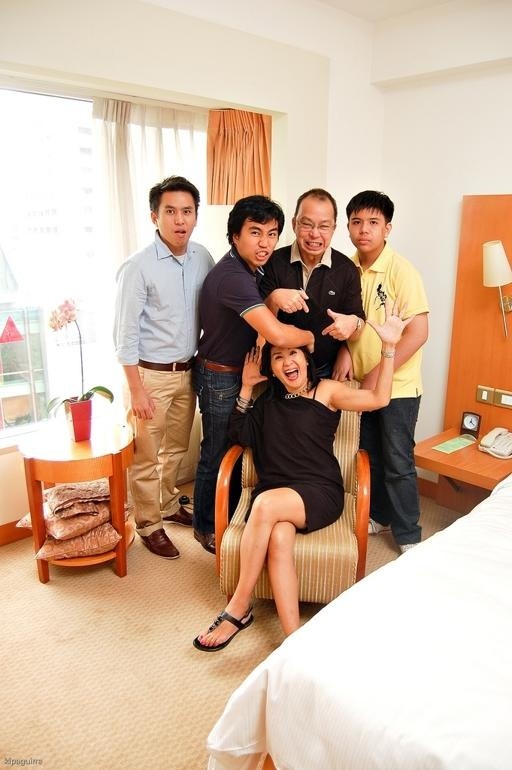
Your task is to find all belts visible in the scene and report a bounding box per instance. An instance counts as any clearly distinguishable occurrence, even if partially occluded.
[137,359,196,372]
[194,353,244,375]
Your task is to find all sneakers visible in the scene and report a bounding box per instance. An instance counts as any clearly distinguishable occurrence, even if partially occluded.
[399,541,419,553]
[366,516,392,535]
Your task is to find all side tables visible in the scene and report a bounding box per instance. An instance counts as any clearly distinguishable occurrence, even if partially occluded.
[17,420,134,581]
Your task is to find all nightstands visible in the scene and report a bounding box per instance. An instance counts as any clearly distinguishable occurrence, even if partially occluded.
[414,428,512,513]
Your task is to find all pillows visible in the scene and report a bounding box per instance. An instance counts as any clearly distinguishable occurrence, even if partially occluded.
[33,511,130,561]
[17,502,128,540]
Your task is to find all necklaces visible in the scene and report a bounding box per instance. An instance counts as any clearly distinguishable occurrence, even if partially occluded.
[285,384,310,399]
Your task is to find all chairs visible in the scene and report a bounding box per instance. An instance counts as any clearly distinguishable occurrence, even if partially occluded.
[214,379,371,604]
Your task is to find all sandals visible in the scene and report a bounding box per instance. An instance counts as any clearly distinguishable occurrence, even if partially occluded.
[192,605,256,653]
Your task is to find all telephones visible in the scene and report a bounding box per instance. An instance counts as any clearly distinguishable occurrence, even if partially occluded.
[478,427,512,456]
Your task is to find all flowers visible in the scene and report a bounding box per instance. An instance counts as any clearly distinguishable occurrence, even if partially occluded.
[47,300,114,416]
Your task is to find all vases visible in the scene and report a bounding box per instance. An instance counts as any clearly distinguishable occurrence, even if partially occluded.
[64,397,92,442]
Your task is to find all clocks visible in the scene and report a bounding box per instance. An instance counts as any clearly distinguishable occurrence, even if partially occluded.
[460,411,481,439]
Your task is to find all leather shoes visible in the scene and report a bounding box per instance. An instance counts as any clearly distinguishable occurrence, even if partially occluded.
[141,526,181,561]
[162,505,194,528]
[194,525,216,554]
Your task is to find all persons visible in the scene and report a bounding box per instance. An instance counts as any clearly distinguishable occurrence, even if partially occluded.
[330,189,430,555]
[190,194,317,555]
[191,293,416,653]
[256,186,368,389]
[112,173,216,561]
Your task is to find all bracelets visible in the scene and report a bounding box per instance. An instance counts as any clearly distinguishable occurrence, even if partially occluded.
[381,350,394,358]
[235,396,254,414]
[357,319,362,335]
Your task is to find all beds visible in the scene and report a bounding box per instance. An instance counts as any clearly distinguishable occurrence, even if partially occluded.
[207,469,512,770]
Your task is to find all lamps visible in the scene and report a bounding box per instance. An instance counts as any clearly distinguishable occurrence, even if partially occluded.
[481,240,512,338]
